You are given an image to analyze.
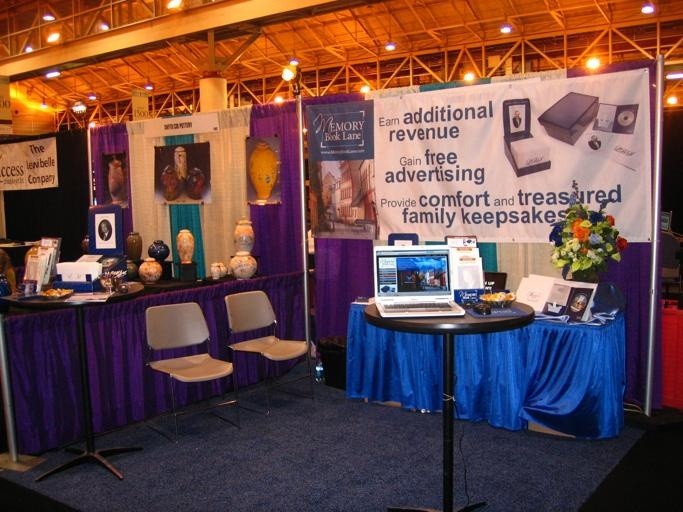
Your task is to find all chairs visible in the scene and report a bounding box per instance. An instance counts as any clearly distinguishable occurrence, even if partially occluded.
[139,303,241,444]
[222,290,307,422]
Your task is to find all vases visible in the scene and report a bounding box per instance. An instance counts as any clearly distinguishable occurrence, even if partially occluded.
[571,268,591,283]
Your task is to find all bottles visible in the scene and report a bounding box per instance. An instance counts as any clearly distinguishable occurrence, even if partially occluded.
[108,159,125,203]
[125,215,258,285]
[247,139,279,200]
[160,146,205,200]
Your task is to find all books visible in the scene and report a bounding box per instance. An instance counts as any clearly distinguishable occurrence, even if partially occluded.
[64,290,116,304]
[20,237,61,293]
[447,235,485,298]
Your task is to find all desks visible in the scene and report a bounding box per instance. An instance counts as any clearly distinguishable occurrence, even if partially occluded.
[3,281,147,484]
[347,295,627,440]
[361,297,534,512]
[1,271,312,459]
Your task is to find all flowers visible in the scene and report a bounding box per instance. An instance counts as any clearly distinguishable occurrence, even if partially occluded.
[548,180,627,281]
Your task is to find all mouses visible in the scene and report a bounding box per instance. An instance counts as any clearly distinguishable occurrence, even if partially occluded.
[473,304,491,315]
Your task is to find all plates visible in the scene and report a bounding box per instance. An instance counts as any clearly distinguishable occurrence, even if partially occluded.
[36,289,74,300]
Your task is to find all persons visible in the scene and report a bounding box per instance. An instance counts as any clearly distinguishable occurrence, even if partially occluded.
[513,111,522,128]
[101,220,112,241]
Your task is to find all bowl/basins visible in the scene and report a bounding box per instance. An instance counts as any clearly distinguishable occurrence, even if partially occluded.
[479,295,516,308]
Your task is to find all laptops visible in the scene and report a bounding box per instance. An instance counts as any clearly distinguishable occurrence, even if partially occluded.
[373,244,466,318]
